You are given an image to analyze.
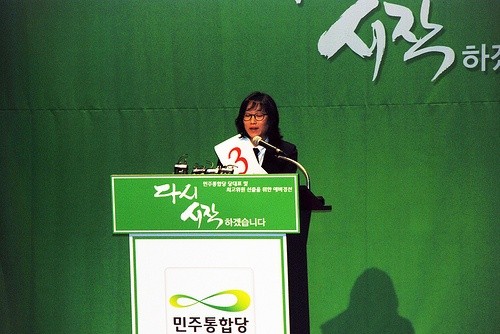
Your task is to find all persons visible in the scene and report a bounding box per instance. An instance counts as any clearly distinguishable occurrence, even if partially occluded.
[217,91,298,174]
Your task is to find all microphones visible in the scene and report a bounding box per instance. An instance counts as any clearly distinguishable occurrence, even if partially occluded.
[253,135,287,156]
[174,154,238,174]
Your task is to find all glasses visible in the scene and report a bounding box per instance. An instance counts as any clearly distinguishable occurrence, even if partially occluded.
[243,112,268,121]
